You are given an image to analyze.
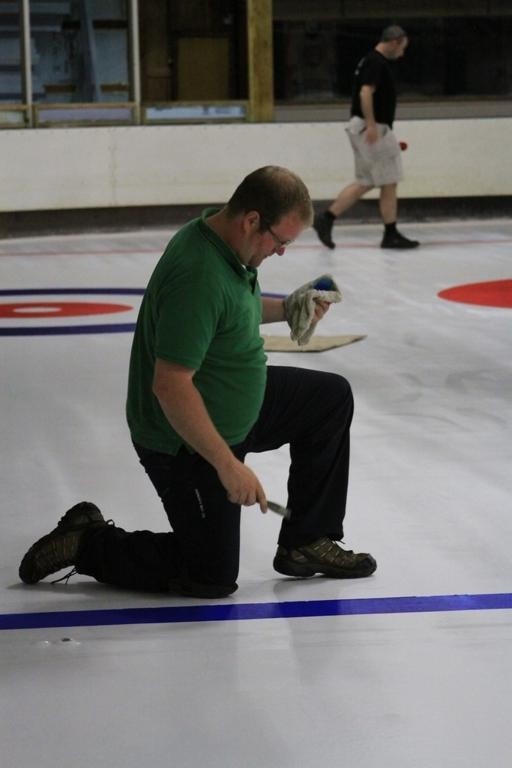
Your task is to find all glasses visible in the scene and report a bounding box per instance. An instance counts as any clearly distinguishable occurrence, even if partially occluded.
[250,208,292,250]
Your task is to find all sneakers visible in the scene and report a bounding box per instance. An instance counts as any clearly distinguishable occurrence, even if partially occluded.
[19,501,114,584]
[381,233,419,249]
[274,535,376,578]
[315,212,336,250]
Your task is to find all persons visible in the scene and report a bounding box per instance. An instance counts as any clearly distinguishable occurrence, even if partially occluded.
[18,166,378,599]
[313,25,420,248]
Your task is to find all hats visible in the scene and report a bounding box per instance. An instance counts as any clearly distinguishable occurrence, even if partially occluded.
[382,25,404,41]
